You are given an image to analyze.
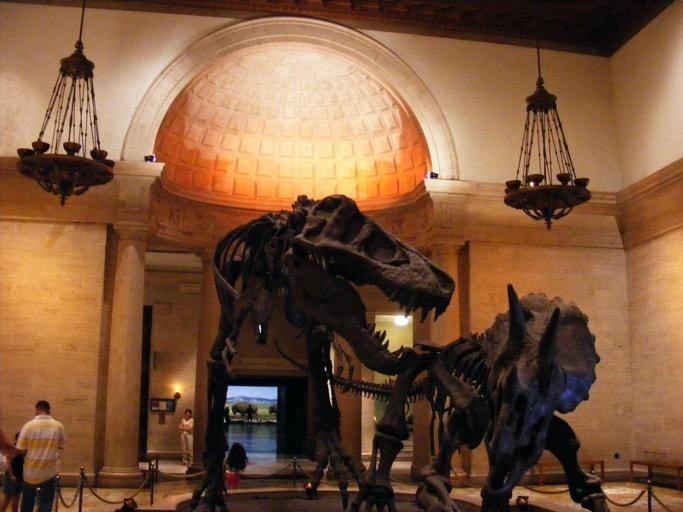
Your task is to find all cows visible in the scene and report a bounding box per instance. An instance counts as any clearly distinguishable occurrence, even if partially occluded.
[268,405,277,415]
[231,403,257,420]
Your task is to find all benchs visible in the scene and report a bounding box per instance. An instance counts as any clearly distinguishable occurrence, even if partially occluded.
[629,455,682,490]
[529,459,605,487]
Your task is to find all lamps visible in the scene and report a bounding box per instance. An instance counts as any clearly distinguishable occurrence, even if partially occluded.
[15,1,115,207]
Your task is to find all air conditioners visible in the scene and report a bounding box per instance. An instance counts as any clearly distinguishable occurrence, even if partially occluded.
[504,21,592,227]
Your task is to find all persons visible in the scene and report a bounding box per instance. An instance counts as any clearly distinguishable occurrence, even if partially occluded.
[246,405,254,425]
[178,409,194,465]
[225,441,248,489]
[14,400,66,512]
[0,430,26,512]
